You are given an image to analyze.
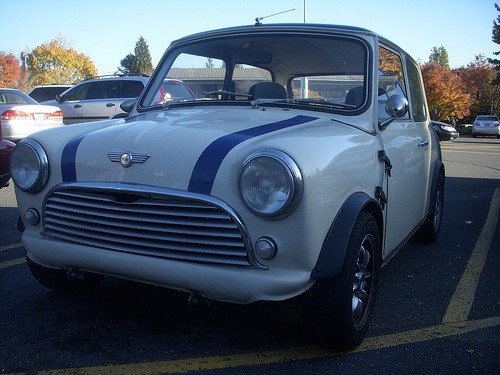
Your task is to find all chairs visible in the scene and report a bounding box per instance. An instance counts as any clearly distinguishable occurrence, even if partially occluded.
[127,82,144,94]
[344,87,387,110]
[84,84,113,99]
[248,81,287,103]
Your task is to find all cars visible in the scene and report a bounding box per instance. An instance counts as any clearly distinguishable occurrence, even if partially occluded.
[471,115,499,138]
[28,84,72,102]
[8,22,447,352]
[458,123,473,128]
[430,119,460,141]
[0,88,65,141]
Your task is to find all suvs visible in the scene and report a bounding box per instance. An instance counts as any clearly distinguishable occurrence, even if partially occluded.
[39,73,196,125]
